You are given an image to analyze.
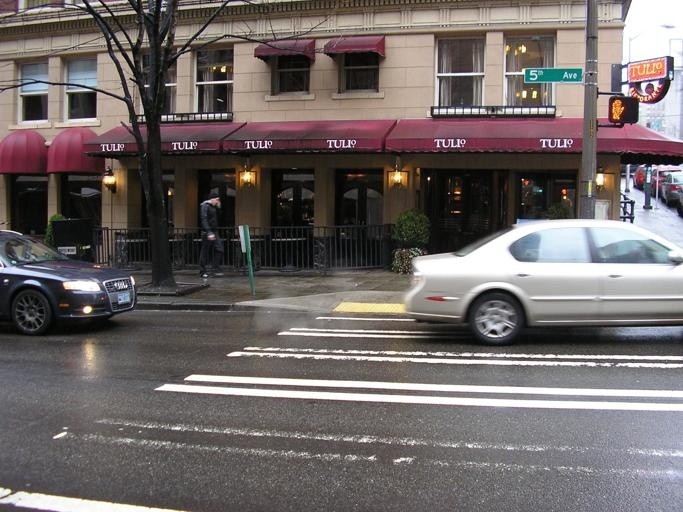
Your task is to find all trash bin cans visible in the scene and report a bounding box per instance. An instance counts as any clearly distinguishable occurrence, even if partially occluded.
[49,219,80,259]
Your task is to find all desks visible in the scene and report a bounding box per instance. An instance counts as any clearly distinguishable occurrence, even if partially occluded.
[115,236,308,271]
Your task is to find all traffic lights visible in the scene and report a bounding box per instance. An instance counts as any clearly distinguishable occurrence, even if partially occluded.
[608,97,638,123]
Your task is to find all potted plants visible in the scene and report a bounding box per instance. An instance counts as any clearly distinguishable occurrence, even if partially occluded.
[392,208,431,272]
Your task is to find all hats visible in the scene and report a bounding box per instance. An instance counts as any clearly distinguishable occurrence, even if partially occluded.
[209,192,218,198]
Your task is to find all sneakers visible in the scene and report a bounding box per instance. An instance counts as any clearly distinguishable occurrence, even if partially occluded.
[200,272,208,278]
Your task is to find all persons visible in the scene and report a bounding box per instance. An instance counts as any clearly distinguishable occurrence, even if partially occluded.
[198,190,226,279]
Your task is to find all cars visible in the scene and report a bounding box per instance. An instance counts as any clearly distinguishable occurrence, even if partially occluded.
[622,160,683,215]
[1,229,136,335]
[406,219,682,347]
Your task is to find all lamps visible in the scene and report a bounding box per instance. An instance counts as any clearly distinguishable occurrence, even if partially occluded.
[388,156,409,192]
[596,164,605,192]
[239,156,257,191]
[454,182,462,194]
[103,165,117,193]
